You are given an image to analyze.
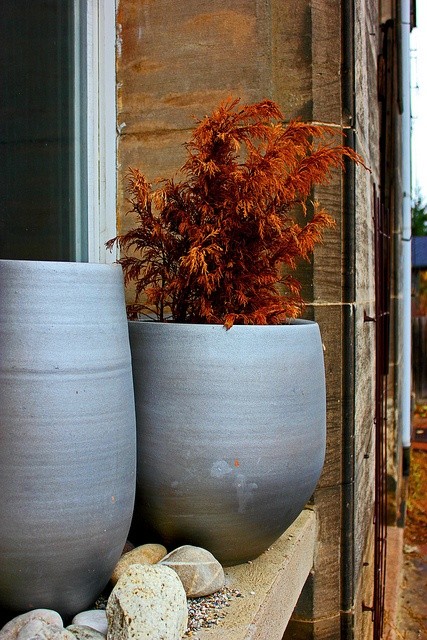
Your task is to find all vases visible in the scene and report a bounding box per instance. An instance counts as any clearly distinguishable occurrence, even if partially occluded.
[0,257,138,620]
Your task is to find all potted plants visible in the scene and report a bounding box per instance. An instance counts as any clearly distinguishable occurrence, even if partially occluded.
[108,94,372,566]
